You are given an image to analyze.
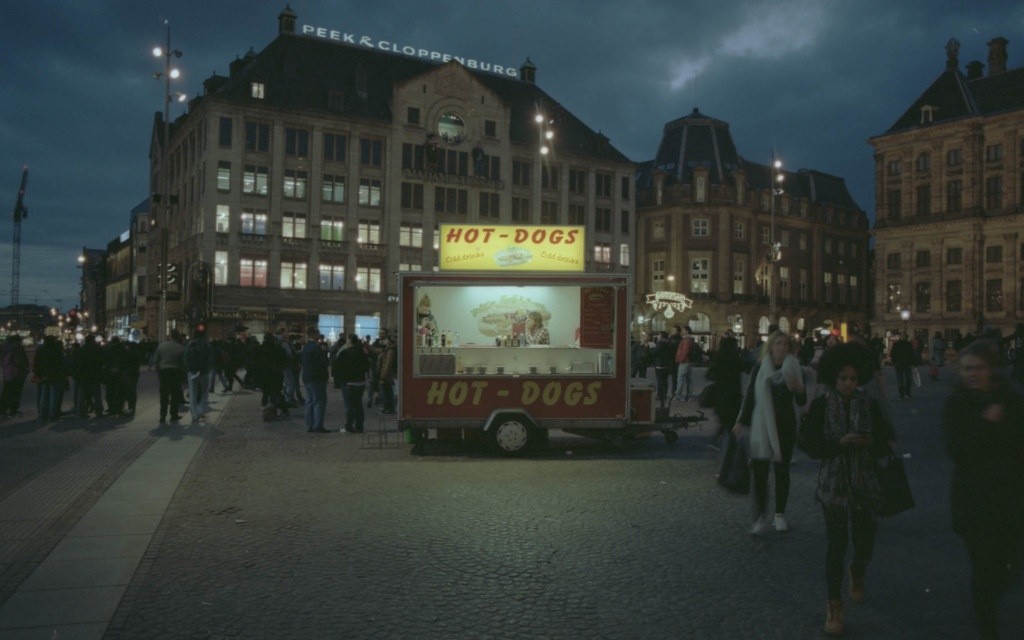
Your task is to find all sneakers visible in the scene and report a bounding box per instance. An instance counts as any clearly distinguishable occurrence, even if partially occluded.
[848,560,865,600]
[825,599,844,635]
[751,513,769,536]
[772,513,788,531]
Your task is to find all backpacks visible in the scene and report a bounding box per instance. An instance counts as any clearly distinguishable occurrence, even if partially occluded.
[681,338,702,362]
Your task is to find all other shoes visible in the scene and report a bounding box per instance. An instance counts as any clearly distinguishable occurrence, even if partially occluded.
[340,429,351,433]
[159,418,166,424]
[171,415,183,421]
[707,441,722,451]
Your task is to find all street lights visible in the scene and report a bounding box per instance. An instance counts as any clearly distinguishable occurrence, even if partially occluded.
[153,20,188,344]
[770,143,785,326]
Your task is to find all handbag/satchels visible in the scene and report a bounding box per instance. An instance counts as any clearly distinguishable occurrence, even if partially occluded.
[261,404,277,422]
[912,367,922,388]
[31,374,44,384]
[872,436,917,517]
[699,385,717,408]
[717,430,751,497]
[929,367,937,376]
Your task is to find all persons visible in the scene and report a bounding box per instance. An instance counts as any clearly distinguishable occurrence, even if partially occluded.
[813,340,901,635]
[731,329,807,535]
[0,323,397,435]
[526,312,550,345]
[941,338,1023,639]
[630,322,1022,487]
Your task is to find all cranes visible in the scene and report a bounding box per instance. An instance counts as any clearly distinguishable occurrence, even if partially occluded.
[10,164,30,312]
